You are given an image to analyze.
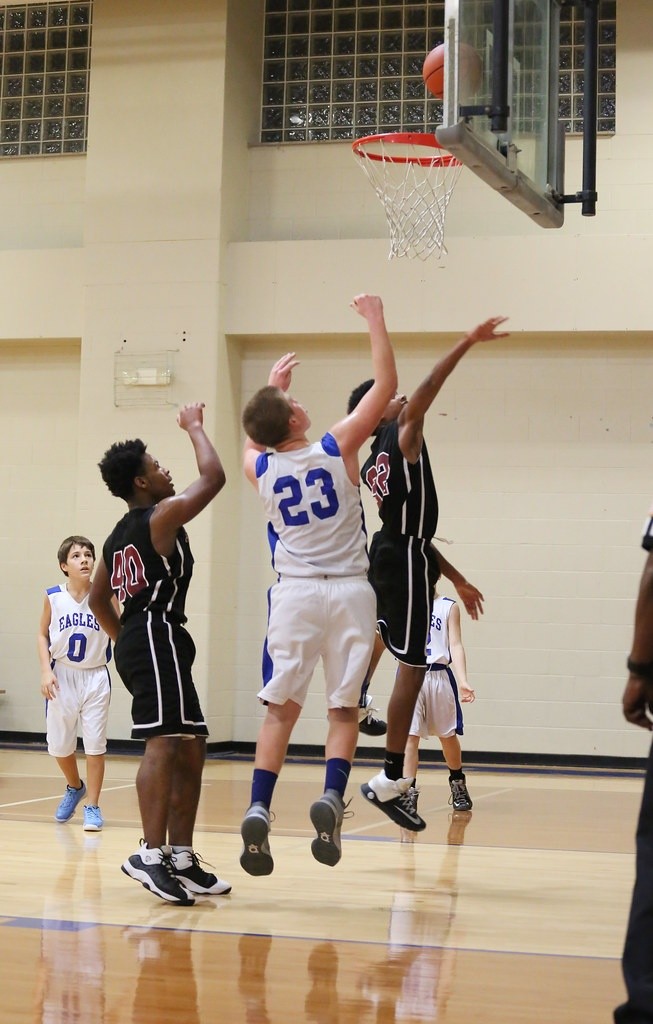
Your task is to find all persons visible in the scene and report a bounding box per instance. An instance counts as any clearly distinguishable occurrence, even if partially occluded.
[36,536,120,835]
[612,512,653,1024]
[240,294,400,876]
[345,315,509,836]
[87,402,233,905]
[401,585,477,811]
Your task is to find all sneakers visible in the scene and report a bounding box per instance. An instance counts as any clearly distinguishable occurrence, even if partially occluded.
[168,847,232,895]
[400,827,417,846]
[83,805,103,831]
[447,772,473,811]
[304,944,338,1020]
[408,786,421,811]
[184,894,231,929]
[122,907,192,948]
[327,694,388,736]
[360,770,426,831]
[240,801,276,876]
[121,837,194,905]
[238,934,272,1004]
[54,780,87,822]
[448,810,472,845]
[309,788,354,867]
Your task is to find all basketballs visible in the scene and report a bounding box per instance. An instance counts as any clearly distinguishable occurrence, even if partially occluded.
[423,42,481,100]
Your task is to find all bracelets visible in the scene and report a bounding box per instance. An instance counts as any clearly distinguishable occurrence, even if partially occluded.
[627,657,653,674]
